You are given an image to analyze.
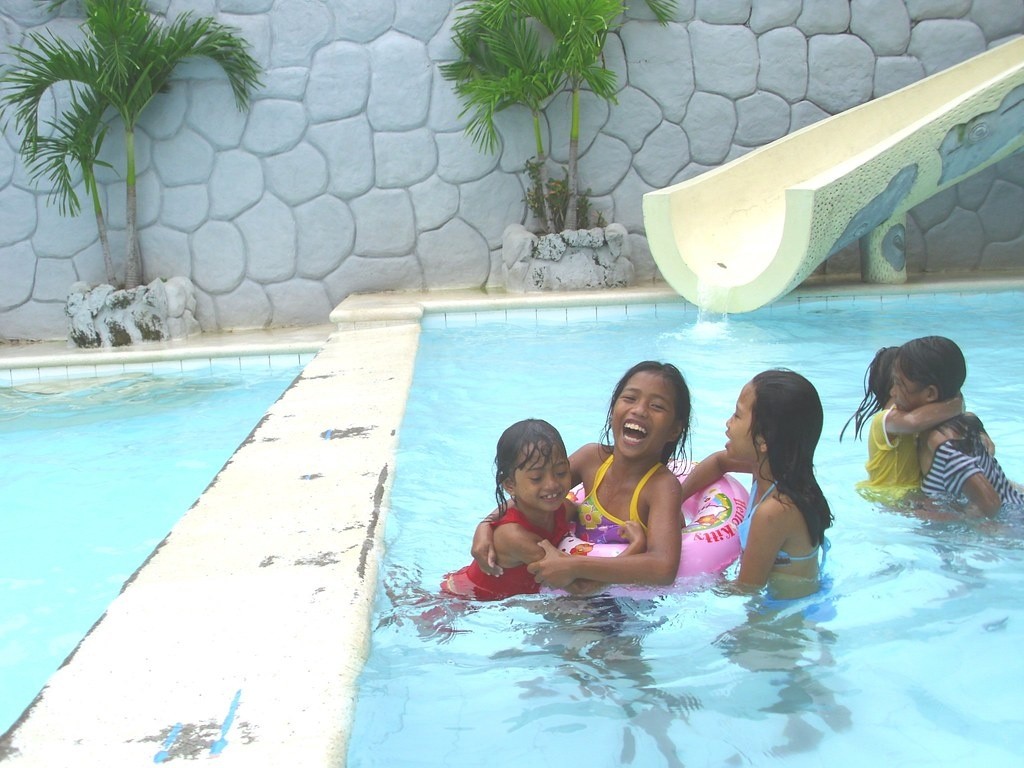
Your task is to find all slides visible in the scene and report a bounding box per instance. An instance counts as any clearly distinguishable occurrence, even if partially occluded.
[641,35,1024,314]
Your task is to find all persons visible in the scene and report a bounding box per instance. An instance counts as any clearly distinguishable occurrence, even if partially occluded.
[470,360,693,591]
[839,335,1024,504]
[681,366,851,585]
[443,418,647,594]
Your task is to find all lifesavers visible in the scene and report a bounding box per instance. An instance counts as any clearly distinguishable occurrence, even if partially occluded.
[543,457,752,594]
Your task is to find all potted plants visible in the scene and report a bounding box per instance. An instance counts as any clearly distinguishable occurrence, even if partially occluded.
[435,1,687,290]
[1,0,268,350]
